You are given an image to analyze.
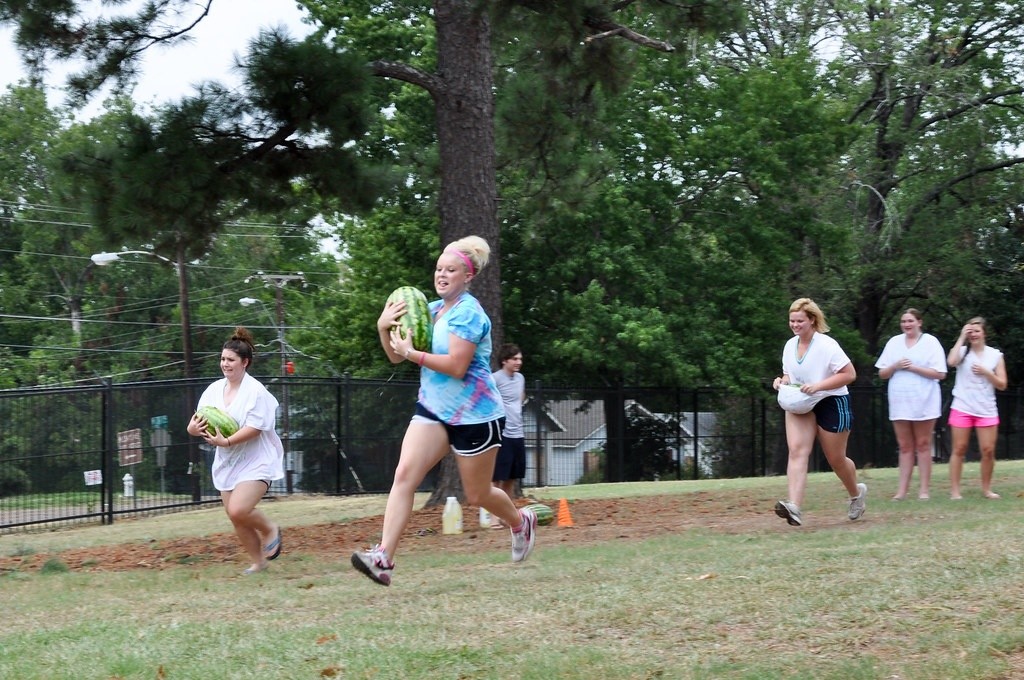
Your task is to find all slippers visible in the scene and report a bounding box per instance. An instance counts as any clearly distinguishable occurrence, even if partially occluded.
[262,522,282,561]
[242,559,269,574]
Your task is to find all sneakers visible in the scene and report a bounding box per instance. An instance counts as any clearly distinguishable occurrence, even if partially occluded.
[846,483,867,522]
[773,498,803,527]
[509,506,538,563]
[351,543,396,587]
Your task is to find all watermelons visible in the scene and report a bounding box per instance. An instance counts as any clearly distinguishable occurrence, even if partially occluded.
[195,406,238,438]
[386,286,432,352]
[522,504,555,526]
[785,383,802,388]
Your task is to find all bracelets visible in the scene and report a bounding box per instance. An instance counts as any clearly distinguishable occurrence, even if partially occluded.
[419,352,426,366]
[226,438,230,446]
[405,347,415,359]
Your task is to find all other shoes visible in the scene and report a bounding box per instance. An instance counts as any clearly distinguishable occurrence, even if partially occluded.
[892,493,904,500]
[951,495,963,500]
[917,489,930,500]
[986,492,1001,499]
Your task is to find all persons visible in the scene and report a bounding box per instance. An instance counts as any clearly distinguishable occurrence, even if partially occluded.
[187,328,284,573]
[947,317,1007,497]
[874,308,947,500]
[773,298,867,526]
[352,235,539,583]
[492,344,525,531]
